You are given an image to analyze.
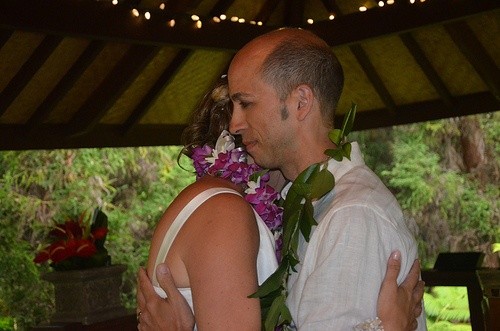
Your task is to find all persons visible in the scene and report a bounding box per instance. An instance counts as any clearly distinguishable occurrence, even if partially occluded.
[135,27,430,331]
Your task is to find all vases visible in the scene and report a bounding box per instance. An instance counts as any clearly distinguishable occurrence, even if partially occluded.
[39,263,130,324]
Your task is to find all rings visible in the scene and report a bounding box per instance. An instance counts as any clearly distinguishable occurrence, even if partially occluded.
[137,312,142,319]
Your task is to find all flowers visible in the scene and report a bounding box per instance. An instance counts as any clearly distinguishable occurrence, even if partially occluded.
[33,206,112,270]
